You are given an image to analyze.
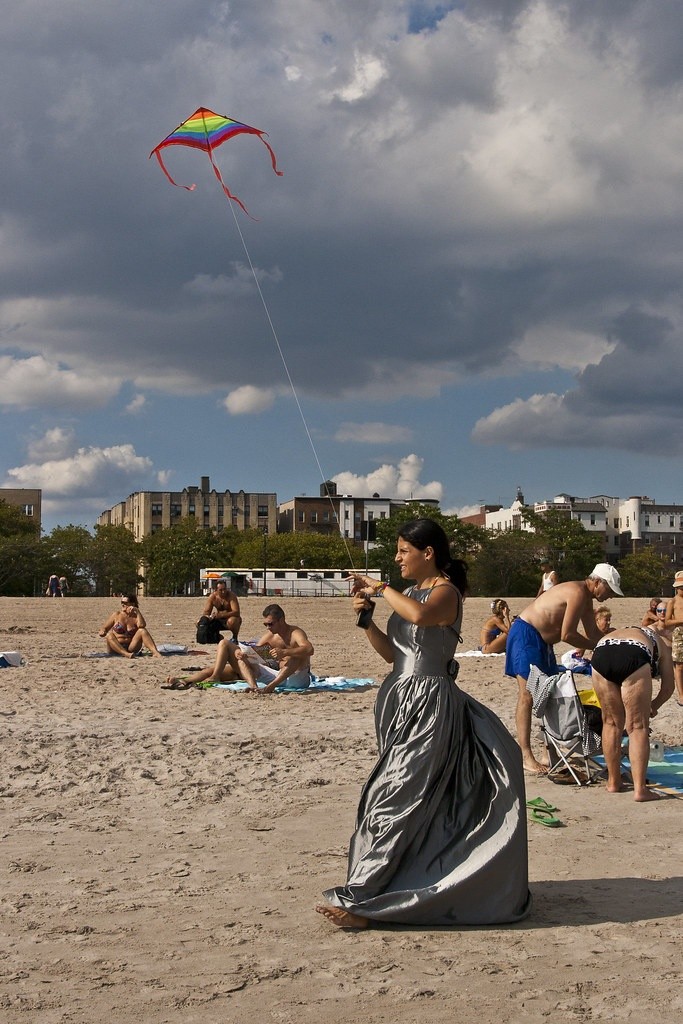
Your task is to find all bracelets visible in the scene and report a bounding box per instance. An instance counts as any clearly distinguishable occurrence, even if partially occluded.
[372,580,387,595]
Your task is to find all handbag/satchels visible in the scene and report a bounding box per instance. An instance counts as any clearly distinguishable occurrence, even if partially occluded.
[46,587,50,596]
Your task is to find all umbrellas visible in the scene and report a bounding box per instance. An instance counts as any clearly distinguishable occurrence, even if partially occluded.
[221,572,238,576]
[203,573,221,578]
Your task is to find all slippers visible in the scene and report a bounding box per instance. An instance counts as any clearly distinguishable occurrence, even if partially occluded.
[548,766,597,783]
[526,797,560,827]
[160,681,189,690]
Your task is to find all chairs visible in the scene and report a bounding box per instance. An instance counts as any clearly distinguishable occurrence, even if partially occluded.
[528,662,650,788]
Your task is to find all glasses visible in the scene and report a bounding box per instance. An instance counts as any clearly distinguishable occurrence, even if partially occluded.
[657,608,666,613]
[263,622,272,626]
[120,601,128,605]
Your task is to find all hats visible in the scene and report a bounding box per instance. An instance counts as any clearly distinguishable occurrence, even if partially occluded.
[539,559,549,564]
[672,570,683,587]
[593,562,624,597]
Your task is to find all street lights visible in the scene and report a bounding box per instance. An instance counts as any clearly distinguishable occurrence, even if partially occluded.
[262,527,268,597]
[365,520,370,577]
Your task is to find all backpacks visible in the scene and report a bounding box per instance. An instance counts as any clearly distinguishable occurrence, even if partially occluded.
[196,616,224,644]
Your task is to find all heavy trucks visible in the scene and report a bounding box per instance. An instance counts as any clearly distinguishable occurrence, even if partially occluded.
[199,568,382,597]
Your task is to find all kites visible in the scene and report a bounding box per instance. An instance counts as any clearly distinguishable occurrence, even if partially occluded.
[148,106,283,222]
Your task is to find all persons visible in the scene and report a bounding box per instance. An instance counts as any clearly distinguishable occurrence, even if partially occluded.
[166,639,244,686]
[641,599,662,627]
[99,594,162,659]
[59,574,69,597]
[576,607,617,659]
[202,580,241,646]
[315,519,531,932]
[665,571,683,706]
[588,625,674,801]
[48,570,59,598]
[505,563,624,774]
[646,602,672,642]
[535,558,558,598]
[234,604,314,694]
[479,599,511,654]
